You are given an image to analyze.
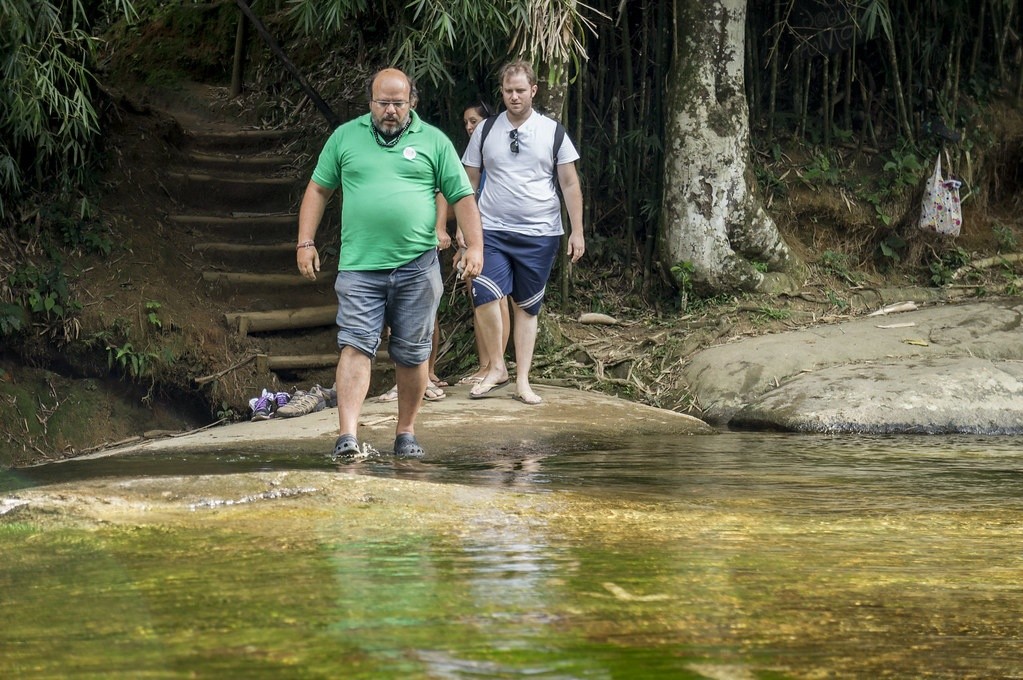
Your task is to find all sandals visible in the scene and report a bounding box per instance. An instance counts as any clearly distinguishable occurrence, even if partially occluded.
[334,434,360,458]
[394,433,425,457]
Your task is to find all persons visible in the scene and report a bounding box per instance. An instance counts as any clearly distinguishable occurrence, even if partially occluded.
[292,68,486,460]
[460,103,513,389]
[452,61,588,404]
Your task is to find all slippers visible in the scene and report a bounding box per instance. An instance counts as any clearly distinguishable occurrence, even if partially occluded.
[421,385,445,402]
[472,378,511,397]
[378,388,400,403]
[430,379,449,387]
[454,376,488,386]
[512,389,541,405]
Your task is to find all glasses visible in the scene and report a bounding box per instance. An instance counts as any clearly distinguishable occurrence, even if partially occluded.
[371,99,410,109]
[508,128,521,154]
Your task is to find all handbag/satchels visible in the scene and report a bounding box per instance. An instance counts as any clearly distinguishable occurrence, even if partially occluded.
[919,149,962,238]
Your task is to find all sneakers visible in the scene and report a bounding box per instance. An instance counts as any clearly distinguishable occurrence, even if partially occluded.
[277,387,326,416]
[274,392,291,411]
[315,384,338,408]
[251,390,276,420]
